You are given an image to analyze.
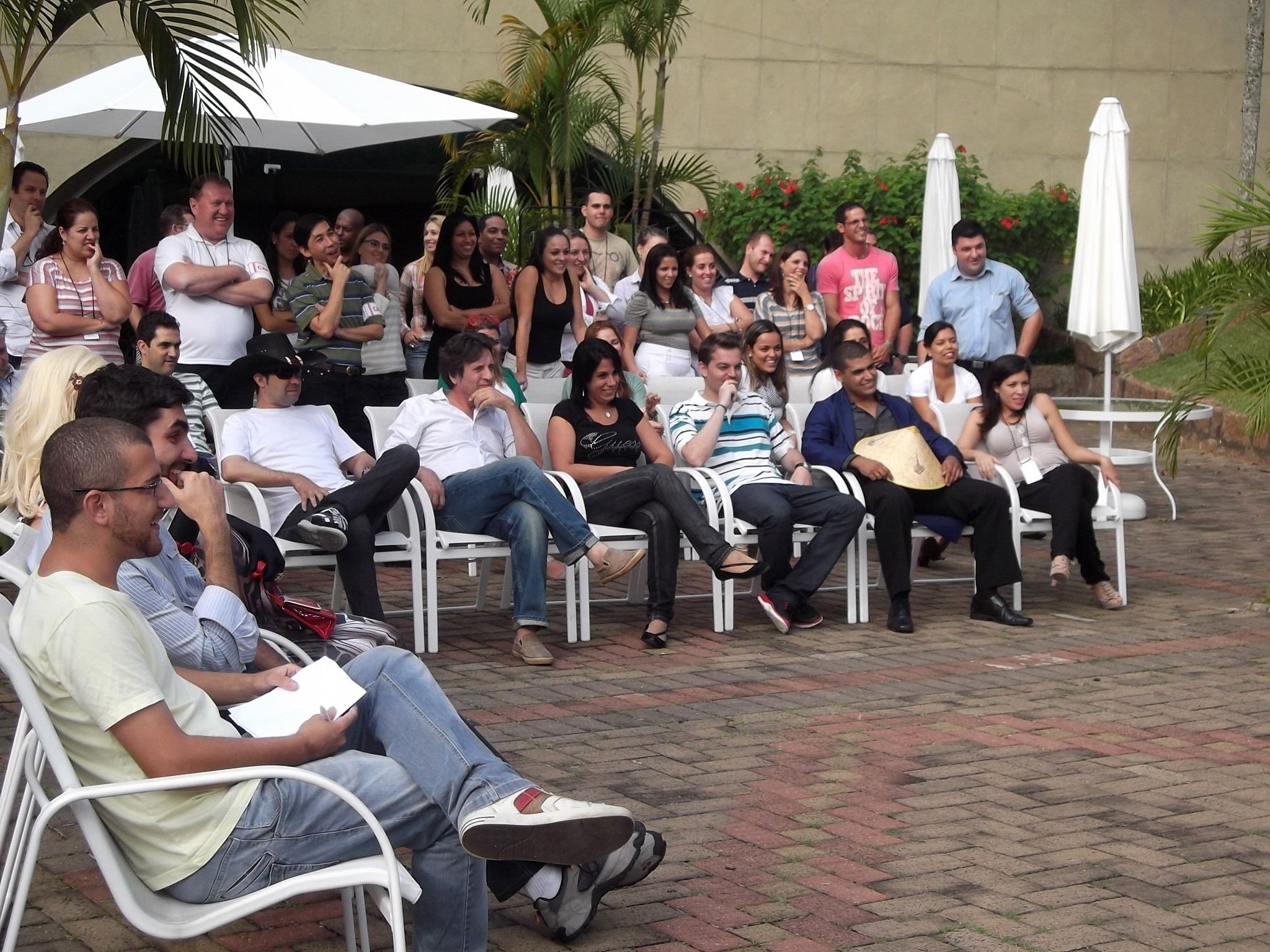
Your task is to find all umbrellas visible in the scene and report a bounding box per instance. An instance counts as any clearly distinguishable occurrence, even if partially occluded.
[1065,93,1144,531]
[0,30,520,254]
[916,129,964,364]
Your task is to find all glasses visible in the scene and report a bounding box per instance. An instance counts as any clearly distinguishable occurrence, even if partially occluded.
[70,478,163,497]
[361,239,392,253]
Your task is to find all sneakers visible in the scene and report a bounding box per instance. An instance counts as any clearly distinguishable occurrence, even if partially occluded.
[533,820,646,942]
[512,633,554,665]
[297,508,348,552]
[594,547,646,584]
[1049,554,1071,584]
[757,586,797,633]
[792,603,823,628]
[458,785,634,865]
[1094,580,1123,610]
[611,830,667,890]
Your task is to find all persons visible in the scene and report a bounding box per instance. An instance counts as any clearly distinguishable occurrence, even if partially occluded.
[801,340,1033,633]
[902,320,985,565]
[668,331,867,635]
[917,218,1048,437]
[217,333,422,616]
[954,354,1125,611]
[9,416,634,952]
[0,159,915,480]
[34,362,667,944]
[379,332,648,668]
[0,344,406,676]
[546,337,771,647]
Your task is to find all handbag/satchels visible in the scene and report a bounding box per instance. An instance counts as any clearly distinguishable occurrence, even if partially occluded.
[250,559,337,640]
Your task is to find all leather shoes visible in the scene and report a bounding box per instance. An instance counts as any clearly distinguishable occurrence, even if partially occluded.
[970,592,1033,625]
[887,604,914,632]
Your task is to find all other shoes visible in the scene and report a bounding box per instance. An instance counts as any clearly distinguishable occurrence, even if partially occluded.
[714,560,771,581]
[641,623,668,648]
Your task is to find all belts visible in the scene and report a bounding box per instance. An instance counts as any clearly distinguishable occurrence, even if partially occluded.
[313,363,366,375]
[956,358,993,370]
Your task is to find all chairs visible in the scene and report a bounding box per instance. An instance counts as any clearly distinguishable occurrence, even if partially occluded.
[0,377,1130,952]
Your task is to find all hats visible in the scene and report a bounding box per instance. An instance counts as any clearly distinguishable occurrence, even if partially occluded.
[224,331,329,401]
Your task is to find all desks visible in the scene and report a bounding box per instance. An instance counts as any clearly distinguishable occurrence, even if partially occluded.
[1043,395,1217,520]
